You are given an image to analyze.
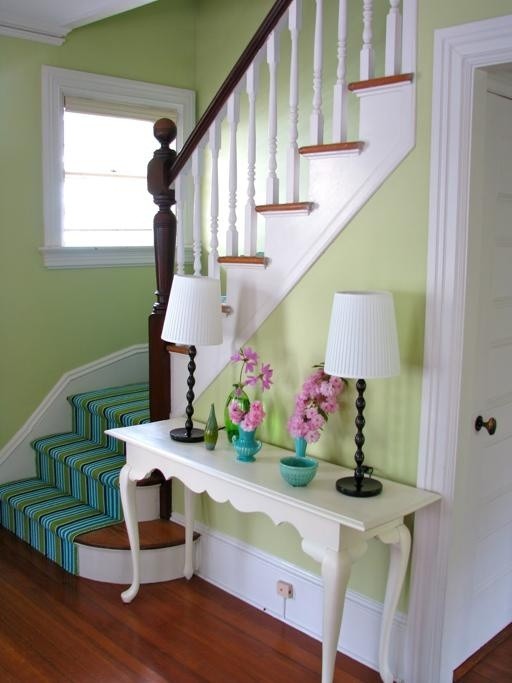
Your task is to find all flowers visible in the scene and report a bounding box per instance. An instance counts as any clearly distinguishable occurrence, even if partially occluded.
[228,346,275,392]
[285,362,348,444]
[227,399,265,431]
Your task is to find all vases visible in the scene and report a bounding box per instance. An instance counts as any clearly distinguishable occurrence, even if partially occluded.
[202,402,219,450]
[281,456,318,486]
[231,427,262,463]
[223,383,251,443]
[294,432,307,459]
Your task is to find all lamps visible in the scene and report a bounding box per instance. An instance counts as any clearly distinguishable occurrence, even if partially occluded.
[160,273,224,444]
[322,290,402,499]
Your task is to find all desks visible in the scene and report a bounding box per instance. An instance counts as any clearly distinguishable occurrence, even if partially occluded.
[102,416,442,683]
